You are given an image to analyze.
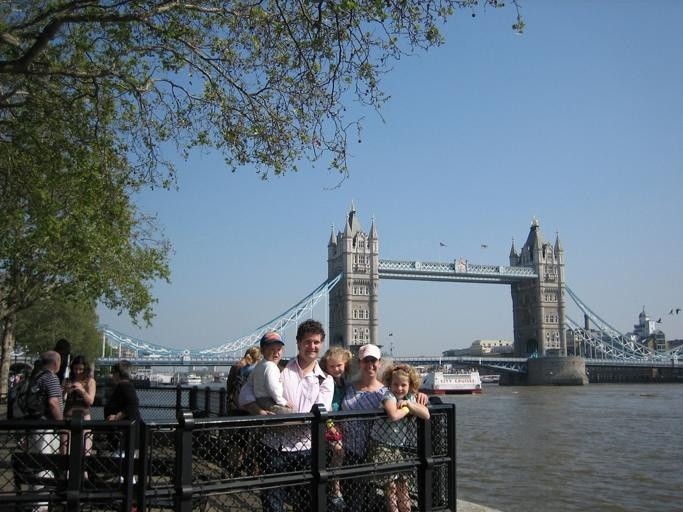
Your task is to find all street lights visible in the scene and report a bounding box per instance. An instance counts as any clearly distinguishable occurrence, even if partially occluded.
[388,332,393,357]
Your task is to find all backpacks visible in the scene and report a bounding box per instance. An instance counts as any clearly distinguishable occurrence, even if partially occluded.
[9,371,52,421]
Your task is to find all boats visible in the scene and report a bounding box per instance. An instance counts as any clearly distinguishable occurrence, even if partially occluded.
[479,375,501,383]
[407,362,482,396]
[133,367,231,387]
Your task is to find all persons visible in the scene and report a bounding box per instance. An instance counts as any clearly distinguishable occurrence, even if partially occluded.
[226,346,262,494]
[338,343,429,512]
[7,336,97,511]
[367,362,430,512]
[315,345,350,508]
[237,318,336,511]
[252,331,296,439]
[102,359,146,511]
[224,348,251,479]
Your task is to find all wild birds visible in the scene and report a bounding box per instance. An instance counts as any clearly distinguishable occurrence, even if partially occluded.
[668,309,673,315]
[676,309,681,314]
[656,318,663,323]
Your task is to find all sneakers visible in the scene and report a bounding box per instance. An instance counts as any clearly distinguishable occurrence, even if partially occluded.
[327,491,346,509]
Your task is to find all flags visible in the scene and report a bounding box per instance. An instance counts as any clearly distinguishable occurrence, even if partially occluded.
[480,244,488,248]
[439,242,448,247]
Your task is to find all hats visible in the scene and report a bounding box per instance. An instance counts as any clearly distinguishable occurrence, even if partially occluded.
[359,344,381,361]
[260,331,284,348]
